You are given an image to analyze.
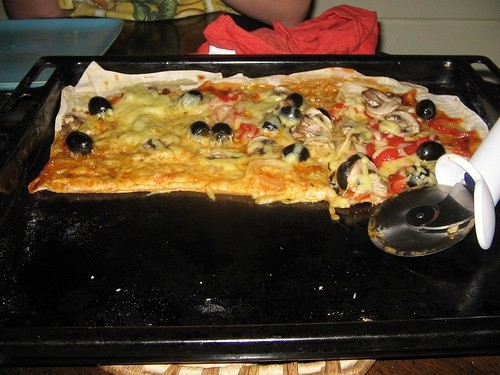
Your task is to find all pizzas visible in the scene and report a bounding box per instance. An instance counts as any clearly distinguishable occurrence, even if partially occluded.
[27,77,484,224]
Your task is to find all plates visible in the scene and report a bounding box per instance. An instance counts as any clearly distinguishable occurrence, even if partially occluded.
[0,15,124,92]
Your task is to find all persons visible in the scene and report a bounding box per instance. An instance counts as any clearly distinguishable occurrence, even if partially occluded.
[2,0,312,27]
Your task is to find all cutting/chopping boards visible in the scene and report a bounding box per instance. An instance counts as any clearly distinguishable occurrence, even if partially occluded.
[0,54,500,368]
